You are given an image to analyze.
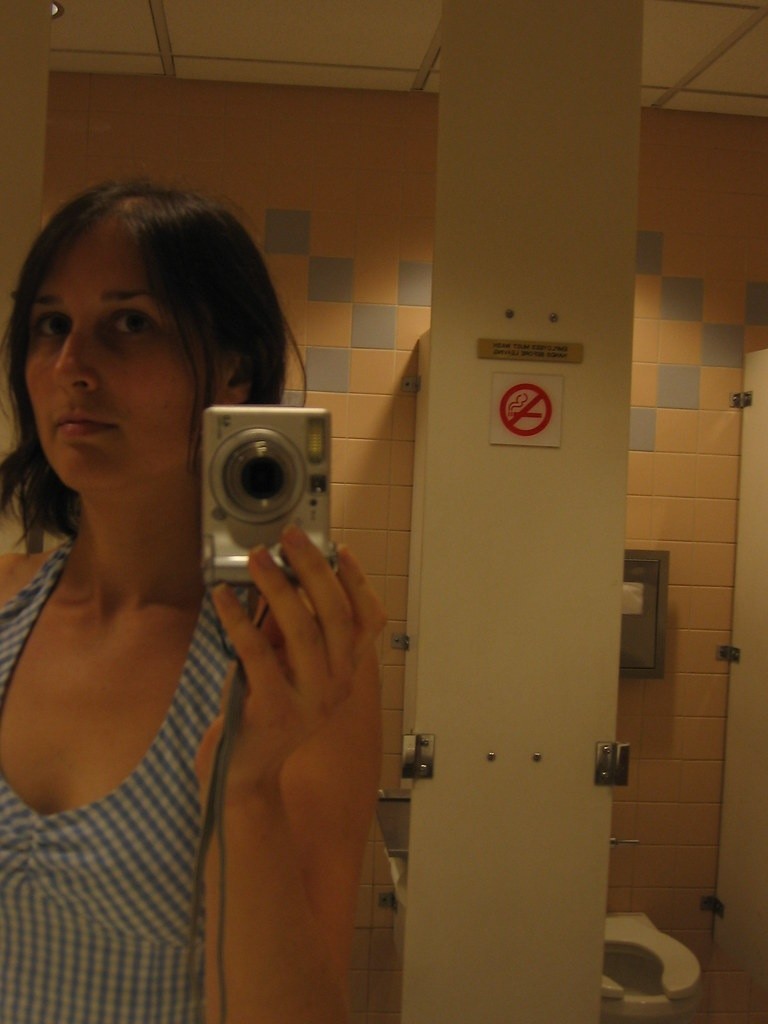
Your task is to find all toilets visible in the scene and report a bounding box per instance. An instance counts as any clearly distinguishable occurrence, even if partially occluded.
[581,911,704,1022]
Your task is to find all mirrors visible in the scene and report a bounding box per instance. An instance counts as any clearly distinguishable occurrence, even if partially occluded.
[0,1,768,1024]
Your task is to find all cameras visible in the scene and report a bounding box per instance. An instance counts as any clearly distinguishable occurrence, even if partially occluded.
[203,402,336,588]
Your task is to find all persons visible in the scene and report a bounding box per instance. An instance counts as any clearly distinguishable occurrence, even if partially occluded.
[0,179,389,1022]
